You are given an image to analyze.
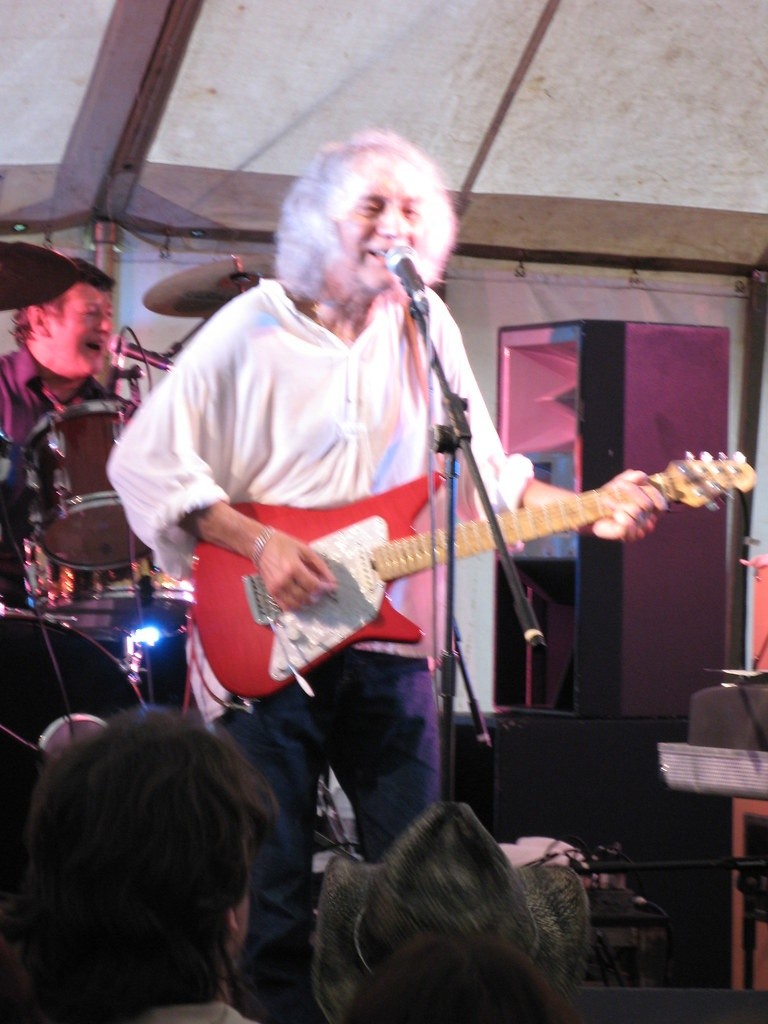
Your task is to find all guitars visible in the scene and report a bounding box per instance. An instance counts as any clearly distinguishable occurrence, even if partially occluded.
[190,447,768,699]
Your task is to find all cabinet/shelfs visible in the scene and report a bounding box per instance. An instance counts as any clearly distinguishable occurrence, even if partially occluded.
[494,318,726,718]
[730,796,768,991]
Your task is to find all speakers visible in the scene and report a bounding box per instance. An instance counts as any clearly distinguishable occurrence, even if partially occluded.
[496,317,745,718]
[453,706,735,987]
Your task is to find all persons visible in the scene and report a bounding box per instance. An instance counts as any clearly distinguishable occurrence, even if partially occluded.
[106,128,667,924]
[1,707,603,1024]
[2,257,134,604]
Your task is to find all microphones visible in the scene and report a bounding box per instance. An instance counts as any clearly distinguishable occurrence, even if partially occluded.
[386,243,429,315]
[103,338,123,401]
[107,335,175,373]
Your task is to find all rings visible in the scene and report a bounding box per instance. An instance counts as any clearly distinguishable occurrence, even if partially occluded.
[637,510,649,524]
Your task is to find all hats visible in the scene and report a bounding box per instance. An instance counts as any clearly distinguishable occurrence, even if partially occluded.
[310,801,590,1024]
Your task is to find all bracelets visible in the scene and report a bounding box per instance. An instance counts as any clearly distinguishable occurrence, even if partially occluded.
[249,526,278,564]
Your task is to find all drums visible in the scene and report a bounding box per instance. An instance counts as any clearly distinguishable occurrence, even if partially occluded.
[0,603,151,822]
[20,398,153,571]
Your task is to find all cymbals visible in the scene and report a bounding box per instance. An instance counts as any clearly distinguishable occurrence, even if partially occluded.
[0,240,80,314]
[141,252,281,318]
